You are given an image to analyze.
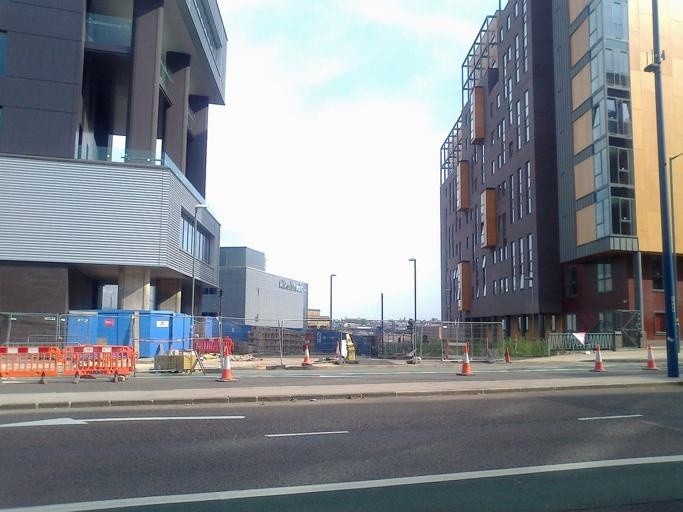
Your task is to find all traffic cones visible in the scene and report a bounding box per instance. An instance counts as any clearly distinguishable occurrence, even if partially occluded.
[216,347,239,383]
[503,347,512,363]
[641,343,658,370]
[456,345,474,376]
[334,342,340,358]
[301,344,312,365]
[589,344,606,372]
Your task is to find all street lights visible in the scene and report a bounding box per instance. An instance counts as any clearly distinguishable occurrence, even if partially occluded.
[328,273,336,329]
[189,203,209,350]
[407,257,416,323]
